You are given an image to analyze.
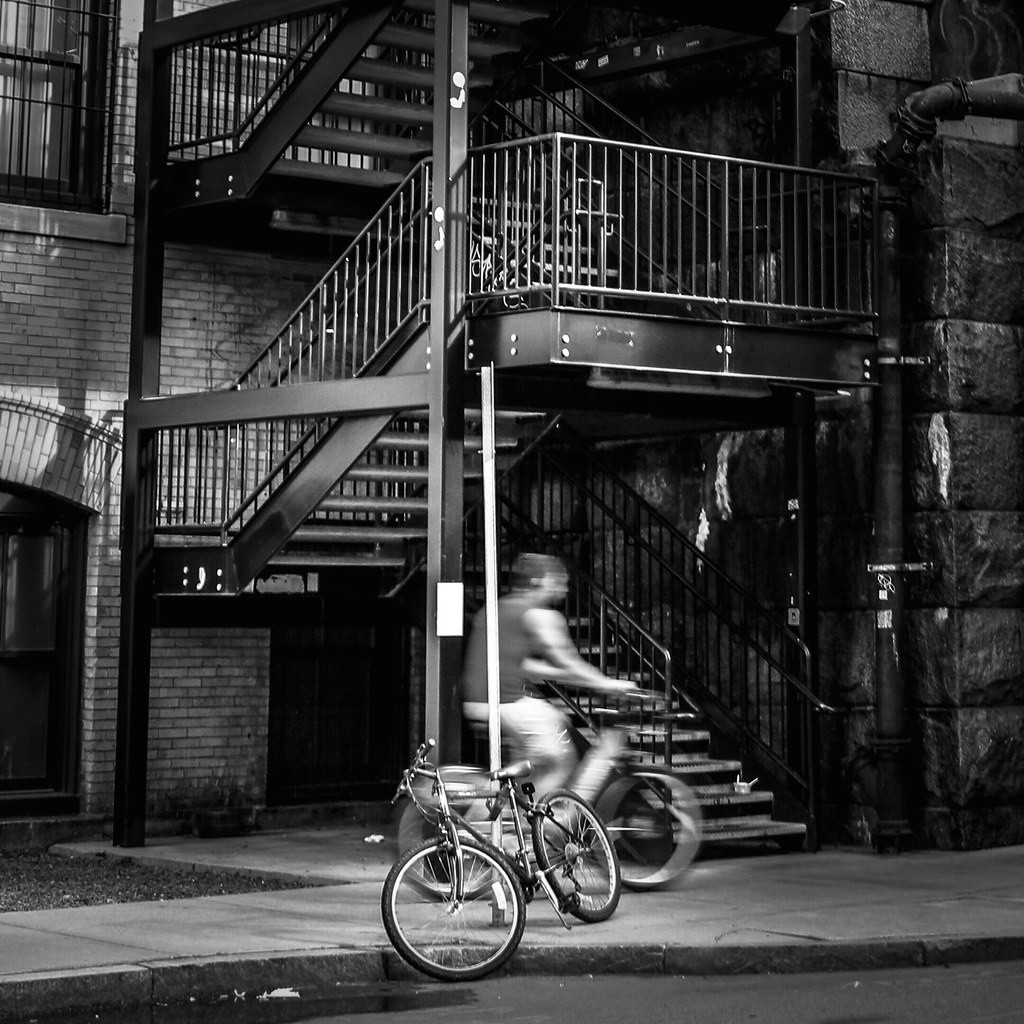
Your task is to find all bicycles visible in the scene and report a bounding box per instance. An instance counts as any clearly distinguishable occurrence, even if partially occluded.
[380,741,624,988]
[403,687,706,897]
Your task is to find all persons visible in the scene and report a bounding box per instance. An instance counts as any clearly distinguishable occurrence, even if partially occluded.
[459,552,637,867]
